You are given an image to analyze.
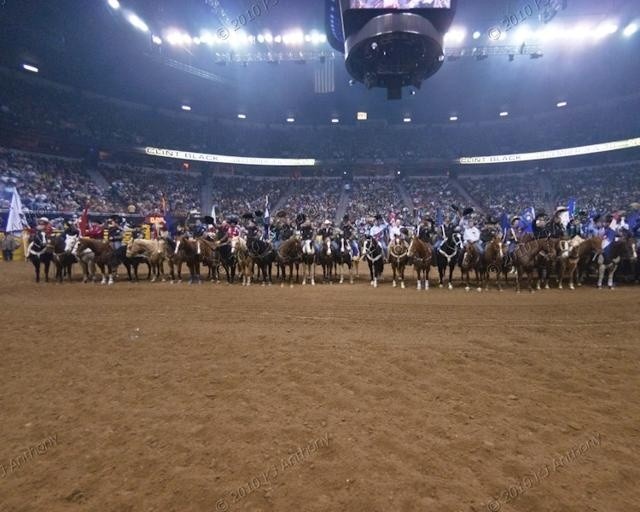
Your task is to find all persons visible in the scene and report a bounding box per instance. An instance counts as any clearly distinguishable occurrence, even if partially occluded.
[1,74,639,284]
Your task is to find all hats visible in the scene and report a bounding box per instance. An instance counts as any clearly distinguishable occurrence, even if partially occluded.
[553,206,567,216]
[486,216,500,225]
[37,217,49,222]
[93,219,102,224]
[510,215,519,225]
[425,218,434,224]
[242,213,254,218]
[535,210,548,219]
[462,207,473,215]
[255,211,263,216]
[204,216,213,224]
[111,215,122,223]
[400,228,408,234]
[323,220,332,225]
[277,211,286,218]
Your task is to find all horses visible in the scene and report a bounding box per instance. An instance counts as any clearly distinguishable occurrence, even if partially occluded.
[26,229,640,296]
